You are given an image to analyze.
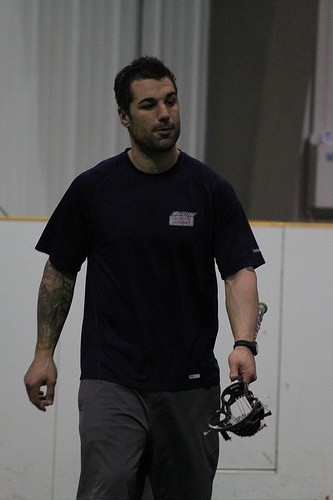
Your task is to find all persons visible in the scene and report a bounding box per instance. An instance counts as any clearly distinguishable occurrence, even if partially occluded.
[24,56,266,500]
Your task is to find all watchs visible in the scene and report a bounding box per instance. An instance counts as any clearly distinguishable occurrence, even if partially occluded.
[233,340,259,356]
